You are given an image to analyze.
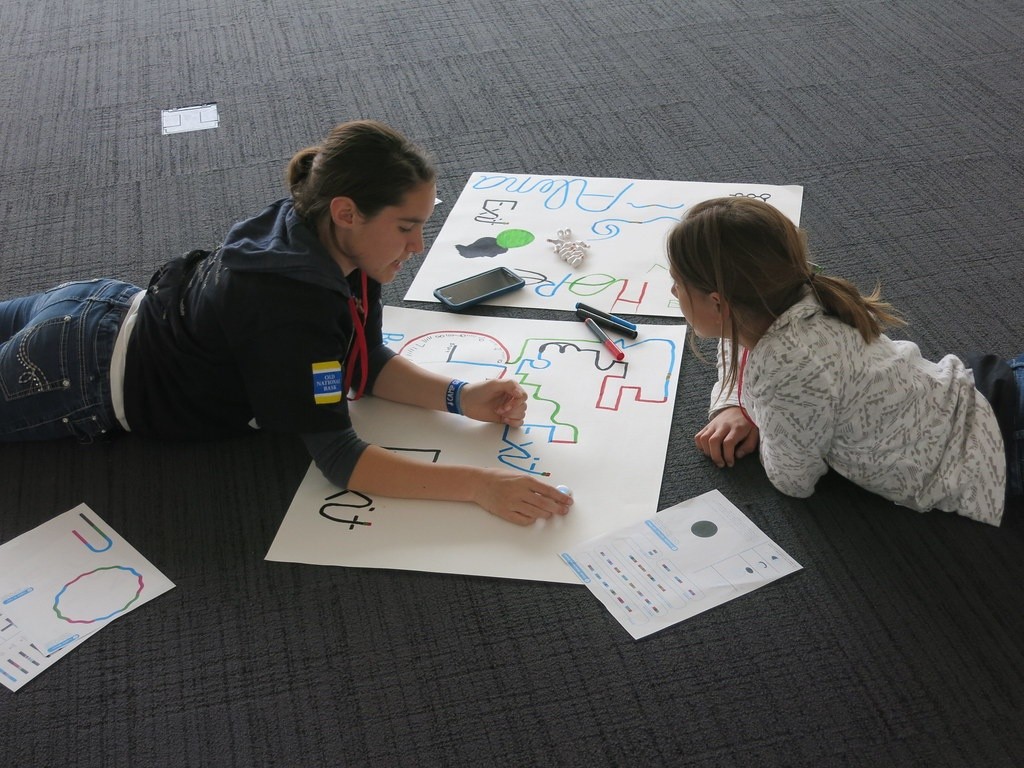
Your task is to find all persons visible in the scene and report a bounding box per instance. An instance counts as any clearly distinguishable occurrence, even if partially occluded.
[666,198,1024,527]
[0,120,573,526]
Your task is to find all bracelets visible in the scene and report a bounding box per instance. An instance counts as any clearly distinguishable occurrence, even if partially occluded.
[445,379,470,415]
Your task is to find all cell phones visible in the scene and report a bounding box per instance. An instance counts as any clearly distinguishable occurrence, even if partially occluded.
[433,267,525,310]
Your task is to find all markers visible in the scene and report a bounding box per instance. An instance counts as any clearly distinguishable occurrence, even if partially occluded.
[585,318,625,361]
[577,308,638,340]
[575,302,637,330]
